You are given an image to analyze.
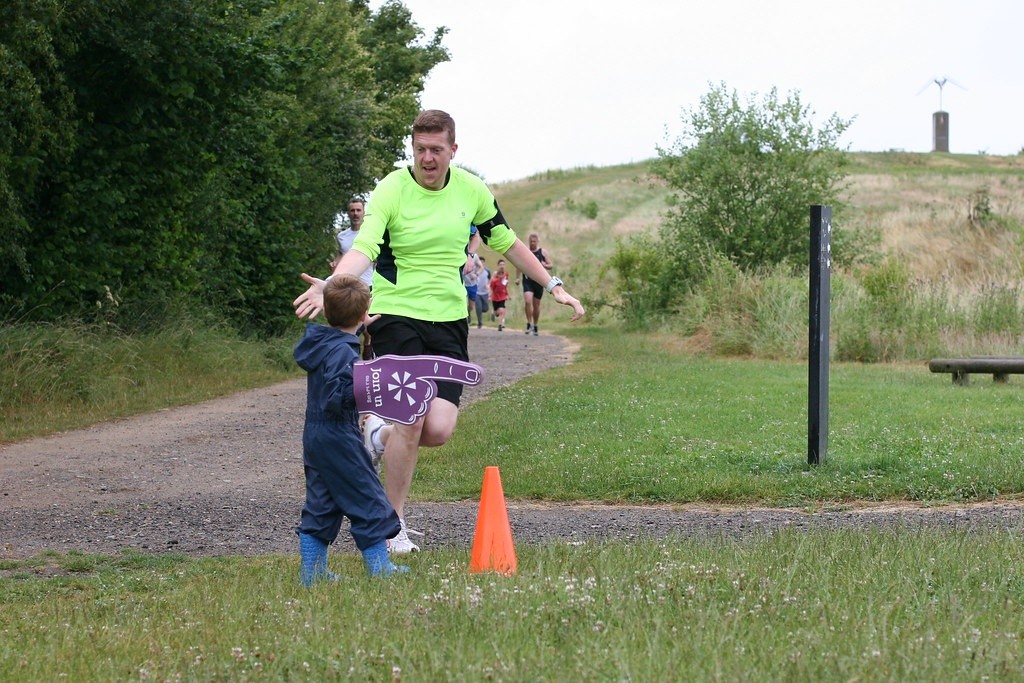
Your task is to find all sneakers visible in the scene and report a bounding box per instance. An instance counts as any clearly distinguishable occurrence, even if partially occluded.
[384,520,425,554]
[361,414,387,477]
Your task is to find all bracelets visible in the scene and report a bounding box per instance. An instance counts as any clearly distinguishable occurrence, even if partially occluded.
[475,273,478,276]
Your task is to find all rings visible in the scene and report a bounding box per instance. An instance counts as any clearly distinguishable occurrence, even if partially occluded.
[468,270,471,272]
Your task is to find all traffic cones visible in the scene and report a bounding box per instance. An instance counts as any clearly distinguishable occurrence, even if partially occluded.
[466,466,517,574]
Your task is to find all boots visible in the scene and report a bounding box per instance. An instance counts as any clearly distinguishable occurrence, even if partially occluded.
[362,538,409,579]
[298,533,337,590]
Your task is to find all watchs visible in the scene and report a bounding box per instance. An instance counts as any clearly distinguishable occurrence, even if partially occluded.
[467,252,474,258]
[545,275,564,293]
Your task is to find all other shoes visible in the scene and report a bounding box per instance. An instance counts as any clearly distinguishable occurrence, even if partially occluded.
[535,326,539,335]
[525,324,532,334]
[498,325,502,331]
[491,313,496,323]
[363,340,376,361]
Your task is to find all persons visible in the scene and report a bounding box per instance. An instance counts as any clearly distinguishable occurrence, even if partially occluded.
[489,259,510,331]
[292,274,482,587]
[292,108,586,556]
[328,196,376,362]
[462,255,484,328]
[514,234,553,336]
[464,221,481,274]
[476,256,491,328]
